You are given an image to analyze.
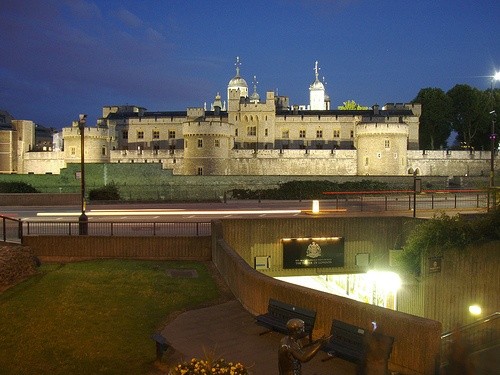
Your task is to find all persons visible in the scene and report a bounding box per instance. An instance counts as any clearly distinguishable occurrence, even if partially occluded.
[443,323,500,375]
[278,318,333,375]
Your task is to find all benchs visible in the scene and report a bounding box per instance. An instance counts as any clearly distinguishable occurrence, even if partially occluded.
[320,319,395,375]
[254,299,319,349]
